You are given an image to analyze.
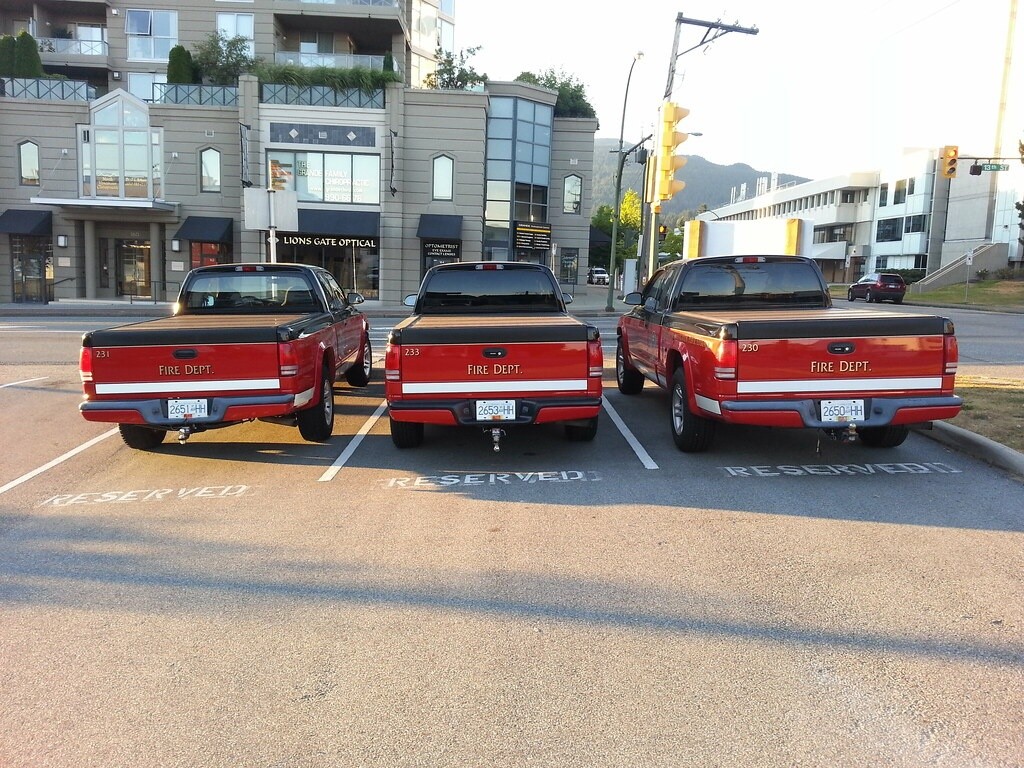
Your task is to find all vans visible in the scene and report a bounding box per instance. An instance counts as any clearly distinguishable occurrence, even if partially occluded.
[591,267,609,285]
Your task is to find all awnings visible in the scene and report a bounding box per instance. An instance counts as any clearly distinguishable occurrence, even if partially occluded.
[0,209,52,235]
[298,209,380,238]
[174,216,233,242]
[416,214,463,239]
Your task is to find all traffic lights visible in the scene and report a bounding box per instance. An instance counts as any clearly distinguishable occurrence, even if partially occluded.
[942,146,958,179]
[659,223,667,241]
[660,103,690,200]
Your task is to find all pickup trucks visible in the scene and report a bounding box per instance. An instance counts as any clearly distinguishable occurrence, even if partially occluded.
[78,264,372,449]
[384,261,604,449]
[615,255,962,453]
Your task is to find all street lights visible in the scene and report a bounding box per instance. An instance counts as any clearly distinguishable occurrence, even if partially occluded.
[605,132,704,311]
[618,52,646,230]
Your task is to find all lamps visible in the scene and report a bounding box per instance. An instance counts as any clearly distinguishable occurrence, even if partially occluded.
[171,239,182,252]
[57,235,67,248]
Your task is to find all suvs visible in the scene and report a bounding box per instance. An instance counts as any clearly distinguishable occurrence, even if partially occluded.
[848,272,906,303]
